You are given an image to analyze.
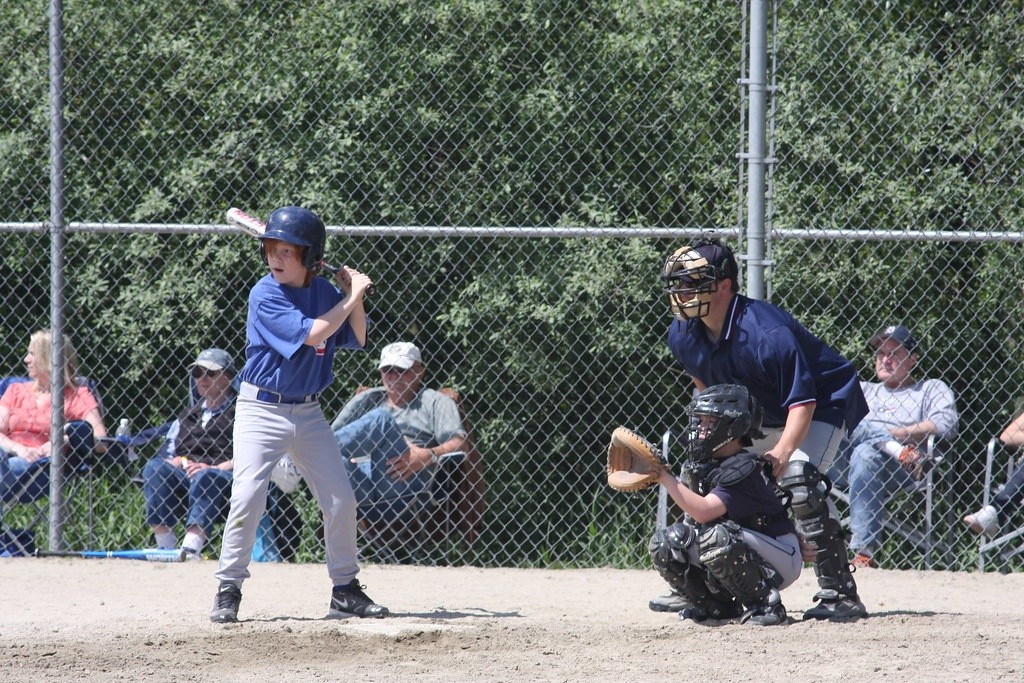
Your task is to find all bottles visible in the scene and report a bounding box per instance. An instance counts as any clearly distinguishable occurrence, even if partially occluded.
[114,418,131,439]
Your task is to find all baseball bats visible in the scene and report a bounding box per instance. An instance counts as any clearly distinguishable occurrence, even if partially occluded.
[225,207,378,296]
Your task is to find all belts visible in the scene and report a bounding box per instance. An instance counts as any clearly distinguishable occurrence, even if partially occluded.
[256,390,315,404]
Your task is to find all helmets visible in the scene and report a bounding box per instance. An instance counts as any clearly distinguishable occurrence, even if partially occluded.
[686,388,769,439]
[255,206,326,267]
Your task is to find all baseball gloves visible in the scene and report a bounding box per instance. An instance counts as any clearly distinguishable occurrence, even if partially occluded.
[606,424,671,493]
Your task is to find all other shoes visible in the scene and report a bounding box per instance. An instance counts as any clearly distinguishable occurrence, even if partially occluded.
[649,592,687,612]
[963,505,999,539]
[899,443,935,481]
[852,556,874,567]
[269,457,302,493]
[803,599,868,619]
[745,604,786,625]
[679,601,744,625]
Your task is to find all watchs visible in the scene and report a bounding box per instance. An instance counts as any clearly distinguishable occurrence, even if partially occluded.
[430,448,439,465]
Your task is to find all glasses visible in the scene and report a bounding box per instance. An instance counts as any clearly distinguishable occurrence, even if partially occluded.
[382,368,407,375]
[192,367,215,377]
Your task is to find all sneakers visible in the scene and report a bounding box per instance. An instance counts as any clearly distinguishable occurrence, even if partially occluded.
[329,579,389,618]
[209,583,242,622]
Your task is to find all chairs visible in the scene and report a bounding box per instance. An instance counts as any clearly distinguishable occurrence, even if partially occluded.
[0,375,1024,573]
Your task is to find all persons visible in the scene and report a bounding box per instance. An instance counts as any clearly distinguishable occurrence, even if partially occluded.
[961,415,1024,538]
[143,348,236,559]
[653,383,801,626]
[649,240,865,623]
[828,325,959,568]
[208,206,389,624]
[0,331,108,472]
[269,342,469,527]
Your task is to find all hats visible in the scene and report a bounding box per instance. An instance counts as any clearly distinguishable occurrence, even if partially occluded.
[378,343,422,370]
[661,244,738,282]
[187,349,235,371]
[870,326,916,358]
